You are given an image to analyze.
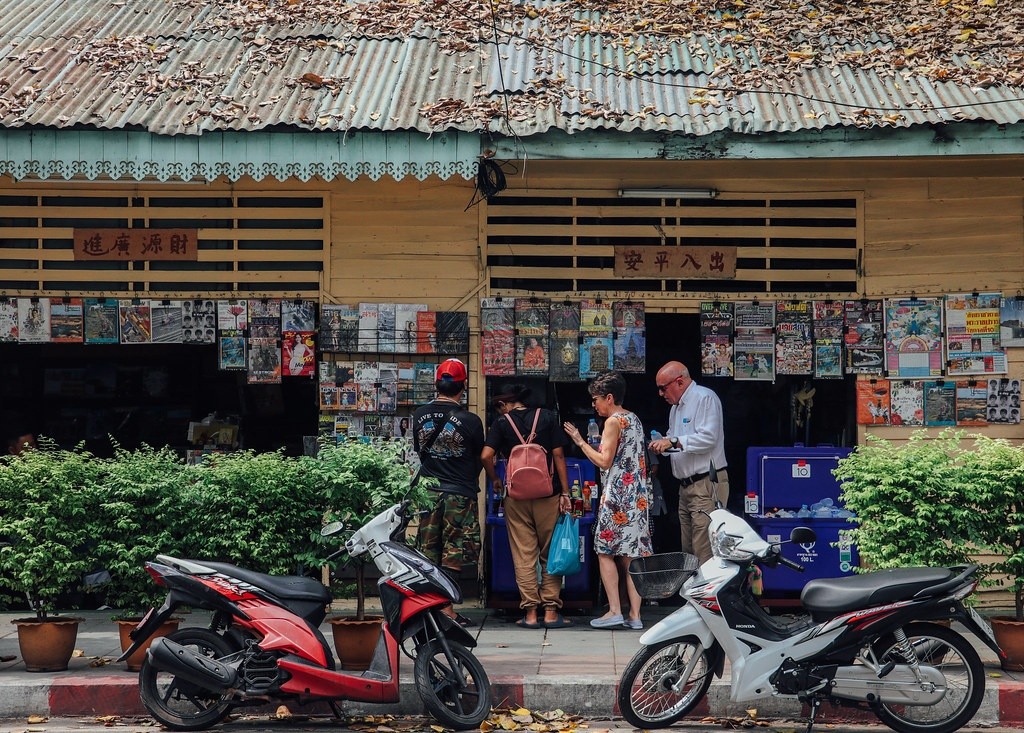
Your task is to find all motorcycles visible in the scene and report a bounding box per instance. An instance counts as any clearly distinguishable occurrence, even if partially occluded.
[618,457,1007,733]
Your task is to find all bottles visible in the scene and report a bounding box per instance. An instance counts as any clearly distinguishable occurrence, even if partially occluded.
[787,497,856,518]
[651,429,670,457]
[582,480,592,511]
[588,418,600,448]
[572,480,581,499]
[493,485,503,514]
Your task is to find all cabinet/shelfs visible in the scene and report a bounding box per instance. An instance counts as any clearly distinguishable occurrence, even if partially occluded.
[744,444,860,616]
[484,456,600,617]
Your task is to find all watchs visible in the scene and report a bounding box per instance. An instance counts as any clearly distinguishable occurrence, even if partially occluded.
[670,437,678,449]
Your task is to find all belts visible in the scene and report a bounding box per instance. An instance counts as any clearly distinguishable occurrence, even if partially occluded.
[680,467,726,488]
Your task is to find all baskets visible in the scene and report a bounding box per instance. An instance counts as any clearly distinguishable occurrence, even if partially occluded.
[627,552,700,599]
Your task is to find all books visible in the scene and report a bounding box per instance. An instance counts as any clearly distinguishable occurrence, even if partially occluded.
[320,302,469,440]
[480,296,645,383]
[0,295,182,343]
[218,298,314,385]
[987,379,1021,424]
[701,295,987,425]
[182,300,216,343]
[1000,296,1024,348]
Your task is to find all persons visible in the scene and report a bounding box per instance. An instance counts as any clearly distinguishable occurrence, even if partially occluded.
[563,369,650,630]
[750,358,759,377]
[526,338,545,367]
[758,355,769,373]
[415,359,484,626]
[737,352,746,372]
[747,354,753,366]
[8,426,34,454]
[182,300,215,342]
[284,333,314,375]
[986,379,1020,423]
[715,344,733,368]
[648,361,728,567]
[399,418,409,437]
[701,344,715,372]
[30,310,40,324]
[481,383,576,629]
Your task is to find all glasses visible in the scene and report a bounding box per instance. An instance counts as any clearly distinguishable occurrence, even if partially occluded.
[657,375,682,393]
[593,393,609,404]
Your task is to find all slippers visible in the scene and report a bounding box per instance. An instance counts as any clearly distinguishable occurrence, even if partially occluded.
[545,614,576,628]
[517,616,541,629]
[453,613,476,627]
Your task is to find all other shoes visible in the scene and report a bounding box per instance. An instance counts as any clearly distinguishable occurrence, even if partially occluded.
[623,618,644,630]
[589,614,624,628]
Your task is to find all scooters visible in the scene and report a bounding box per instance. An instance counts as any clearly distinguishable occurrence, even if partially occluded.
[115,465,492,731]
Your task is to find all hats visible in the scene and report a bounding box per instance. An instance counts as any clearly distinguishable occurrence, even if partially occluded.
[436,358,467,383]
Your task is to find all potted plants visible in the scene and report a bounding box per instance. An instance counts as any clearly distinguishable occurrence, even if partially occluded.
[826,426,1024,673]
[0,424,442,674]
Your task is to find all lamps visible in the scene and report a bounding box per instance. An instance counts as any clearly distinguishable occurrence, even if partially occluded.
[618,188,721,199]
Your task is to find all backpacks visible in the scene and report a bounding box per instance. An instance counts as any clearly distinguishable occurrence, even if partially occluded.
[504,408,555,500]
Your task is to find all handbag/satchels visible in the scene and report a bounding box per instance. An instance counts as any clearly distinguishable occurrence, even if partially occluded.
[545,512,581,575]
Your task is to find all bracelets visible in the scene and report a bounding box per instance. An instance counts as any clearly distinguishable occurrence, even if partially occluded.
[561,494,569,496]
